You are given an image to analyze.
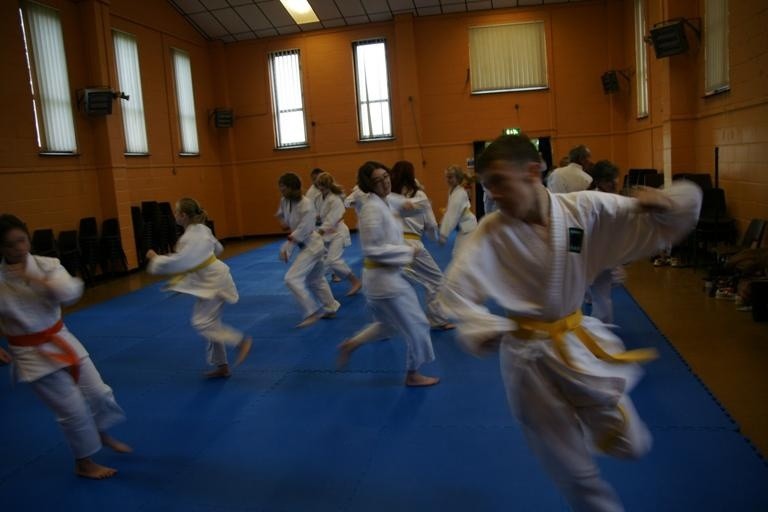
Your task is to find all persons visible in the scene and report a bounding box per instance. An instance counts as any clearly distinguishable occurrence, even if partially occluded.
[442,163,477,259]
[585,158,622,331]
[314,172,362,297]
[0,213,134,480]
[546,144,594,193]
[435,135,703,512]
[272,173,340,328]
[337,161,441,387]
[388,161,456,330]
[146,196,251,380]
[345,183,370,228]
[305,168,342,282]
[481,174,501,215]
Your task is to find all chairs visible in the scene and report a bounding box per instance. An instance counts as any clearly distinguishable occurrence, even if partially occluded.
[100,218,128,280]
[79,217,109,288]
[31,229,55,256]
[131,201,214,269]
[620,169,664,196]
[707,219,767,303]
[672,173,735,263]
[58,230,93,290]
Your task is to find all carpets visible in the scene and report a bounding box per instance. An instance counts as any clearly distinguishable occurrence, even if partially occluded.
[1,229,767,510]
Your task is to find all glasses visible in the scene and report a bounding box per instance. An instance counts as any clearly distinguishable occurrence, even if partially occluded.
[370,173,392,187]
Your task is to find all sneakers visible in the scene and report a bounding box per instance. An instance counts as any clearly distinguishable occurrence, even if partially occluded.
[716,279,732,288]
[650,253,689,267]
[735,294,752,312]
[715,287,738,301]
[705,280,716,297]
